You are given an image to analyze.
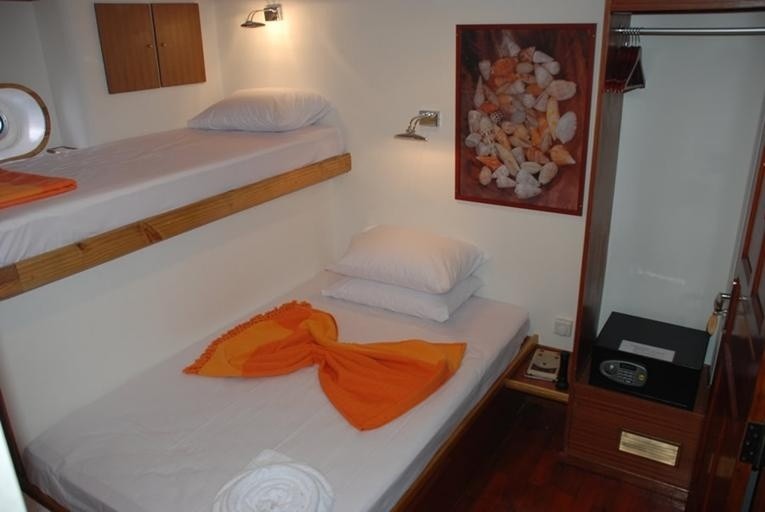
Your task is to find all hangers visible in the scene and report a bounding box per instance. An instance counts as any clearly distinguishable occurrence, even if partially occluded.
[603,26,645,94]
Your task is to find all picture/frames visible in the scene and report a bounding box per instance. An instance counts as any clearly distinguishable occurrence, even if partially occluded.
[454,23,596,216]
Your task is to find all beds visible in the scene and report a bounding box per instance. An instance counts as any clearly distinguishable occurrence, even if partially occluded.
[0,125,352,301]
[23,270,539,512]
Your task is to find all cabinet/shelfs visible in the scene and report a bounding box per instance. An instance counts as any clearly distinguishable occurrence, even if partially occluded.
[559,0,765,503]
[94,3,206,94]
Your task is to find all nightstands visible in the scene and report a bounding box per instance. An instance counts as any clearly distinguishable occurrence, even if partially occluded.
[504,343,573,407]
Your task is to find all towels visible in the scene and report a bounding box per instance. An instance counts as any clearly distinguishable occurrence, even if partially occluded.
[210,448,335,512]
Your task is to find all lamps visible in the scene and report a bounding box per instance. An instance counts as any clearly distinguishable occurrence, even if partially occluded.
[394,111,439,141]
[241,4,282,28]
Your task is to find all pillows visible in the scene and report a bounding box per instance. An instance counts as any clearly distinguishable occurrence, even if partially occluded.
[321,225,492,324]
[187,87,331,132]
